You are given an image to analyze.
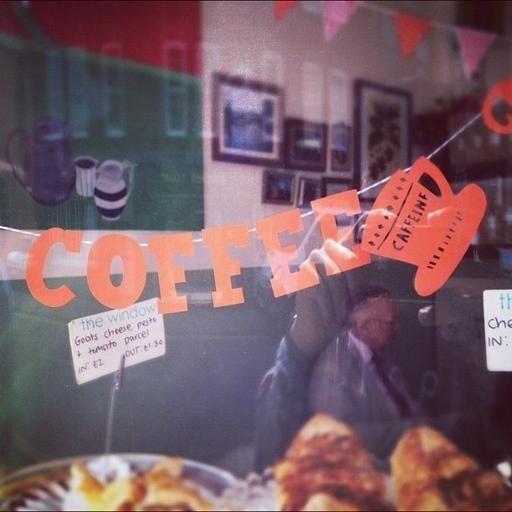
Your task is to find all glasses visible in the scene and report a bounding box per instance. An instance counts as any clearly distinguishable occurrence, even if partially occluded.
[365,315,399,326]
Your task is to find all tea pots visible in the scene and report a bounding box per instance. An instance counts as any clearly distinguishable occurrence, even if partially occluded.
[96,158,136,221]
[7,116,74,207]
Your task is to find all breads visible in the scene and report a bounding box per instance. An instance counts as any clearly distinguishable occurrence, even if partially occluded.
[69,412,512,512]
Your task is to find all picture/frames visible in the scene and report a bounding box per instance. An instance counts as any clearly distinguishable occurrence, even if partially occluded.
[210,70,416,242]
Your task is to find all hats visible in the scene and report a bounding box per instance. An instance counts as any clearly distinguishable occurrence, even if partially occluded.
[343,284,393,312]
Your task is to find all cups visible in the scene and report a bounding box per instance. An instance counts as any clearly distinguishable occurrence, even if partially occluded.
[71,153,99,200]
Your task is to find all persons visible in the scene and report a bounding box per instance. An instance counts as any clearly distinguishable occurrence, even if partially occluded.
[252,238,358,470]
[305,286,475,461]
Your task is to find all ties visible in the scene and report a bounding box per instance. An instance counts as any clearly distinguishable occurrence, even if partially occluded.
[367,355,414,422]
